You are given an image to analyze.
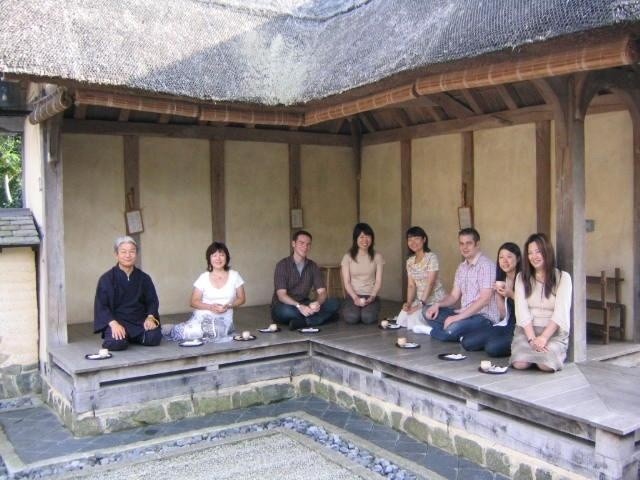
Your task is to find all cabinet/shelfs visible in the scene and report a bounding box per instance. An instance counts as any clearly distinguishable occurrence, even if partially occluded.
[586,267,625,343]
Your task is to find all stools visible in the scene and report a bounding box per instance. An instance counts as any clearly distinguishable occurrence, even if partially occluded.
[319,264,345,303]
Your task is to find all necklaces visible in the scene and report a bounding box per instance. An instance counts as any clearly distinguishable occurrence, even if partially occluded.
[219,277,222,280]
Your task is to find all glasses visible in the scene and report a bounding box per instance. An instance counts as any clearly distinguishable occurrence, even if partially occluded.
[118,249,136,255]
[294,241,313,250]
[210,253,226,259]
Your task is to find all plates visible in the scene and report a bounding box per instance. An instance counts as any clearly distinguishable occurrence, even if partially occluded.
[234,336,256,342]
[378,324,401,329]
[478,365,508,374]
[259,328,282,333]
[395,342,422,349]
[438,353,467,360]
[298,327,319,333]
[178,339,203,347]
[84,354,113,360]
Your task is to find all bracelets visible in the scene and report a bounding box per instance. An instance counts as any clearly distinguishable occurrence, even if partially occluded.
[420,300,426,306]
[528,337,536,343]
[144,317,160,327]
[296,302,303,309]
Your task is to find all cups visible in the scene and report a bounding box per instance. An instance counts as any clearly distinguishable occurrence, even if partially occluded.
[309,302,319,312]
[496,281,505,291]
[241,331,250,341]
[359,298,367,306]
[481,360,492,373]
[381,320,388,329]
[99,349,109,358]
[397,337,407,348]
[269,324,277,332]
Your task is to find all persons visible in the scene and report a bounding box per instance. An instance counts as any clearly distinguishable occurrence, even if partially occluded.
[93,236,162,351]
[411,228,501,342]
[341,223,386,325]
[462,242,523,357]
[271,231,341,330]
[396,226,450,327]
[508,233,572,372]
[161,242,246,341]
